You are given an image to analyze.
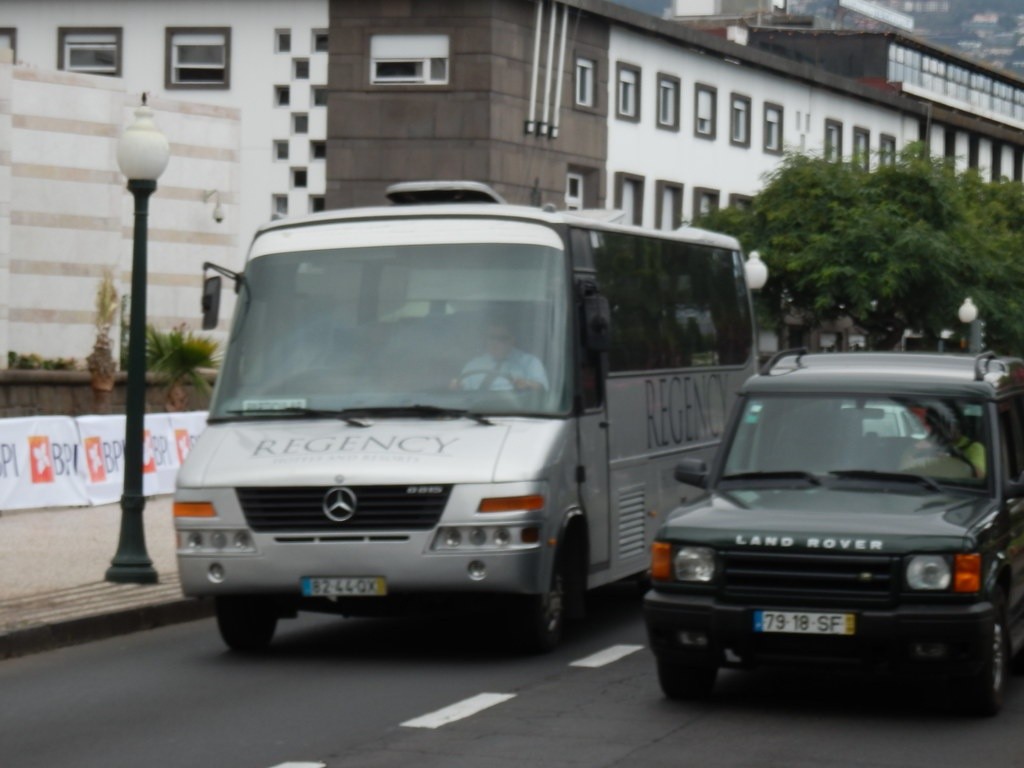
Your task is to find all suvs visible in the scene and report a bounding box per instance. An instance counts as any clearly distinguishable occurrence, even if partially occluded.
[644,347,1024,716]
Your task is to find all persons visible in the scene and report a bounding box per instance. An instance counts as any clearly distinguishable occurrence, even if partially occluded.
[445,316,551,396]
[903,402,988,488]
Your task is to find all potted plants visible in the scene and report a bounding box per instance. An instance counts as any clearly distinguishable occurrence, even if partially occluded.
[88,275,120,391]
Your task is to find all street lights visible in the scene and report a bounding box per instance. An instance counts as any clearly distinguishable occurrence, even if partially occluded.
[103,91,172,583]
[745,250,768,303]
[959,298,978,352]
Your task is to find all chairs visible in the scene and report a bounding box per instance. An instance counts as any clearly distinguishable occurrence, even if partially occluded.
[775,410,864,480]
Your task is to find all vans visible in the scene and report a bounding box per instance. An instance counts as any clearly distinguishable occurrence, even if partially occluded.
[174,180,759,654]
